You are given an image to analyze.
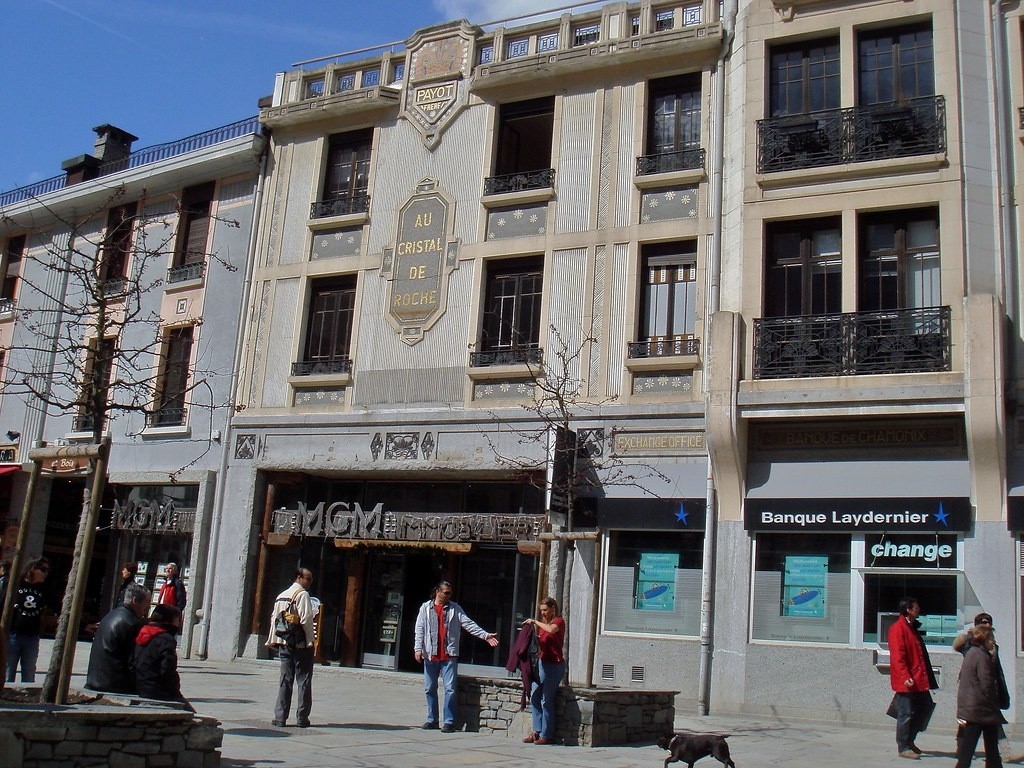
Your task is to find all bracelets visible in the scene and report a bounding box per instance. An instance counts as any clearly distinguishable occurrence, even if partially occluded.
[531,620,535,624]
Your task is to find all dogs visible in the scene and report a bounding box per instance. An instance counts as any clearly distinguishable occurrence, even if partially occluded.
[651,731,737,767]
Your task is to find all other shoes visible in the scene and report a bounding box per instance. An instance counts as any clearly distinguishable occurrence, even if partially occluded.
[899,749,920,759]
[297,719,310,727]
[534,737,555,745]
[523,731,539,743]
[911,744,921,754]
[422,721,439,729]
[272,718,286,727]
[441,723,456,732]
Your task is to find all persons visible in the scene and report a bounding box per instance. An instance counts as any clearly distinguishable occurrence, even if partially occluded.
[265,568,317,727]
[953,613,1010,768]
[86,584,152,693]
[415,581,499,732]
[117,563,138,609]
[521,597,566,745]
[4,553,102,682]
[888,599,939,759]
[135,604,197,714]
[158,563,186,611]
[0,563,9,612]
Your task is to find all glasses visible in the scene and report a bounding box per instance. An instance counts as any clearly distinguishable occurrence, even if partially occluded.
[441,590,450,595]
[35,565,49,573]
[303,578,312,581]
[981,621,992,625]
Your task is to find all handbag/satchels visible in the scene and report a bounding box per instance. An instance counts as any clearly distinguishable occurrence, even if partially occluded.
[887,692,936,732]
[275,589,307,650]
